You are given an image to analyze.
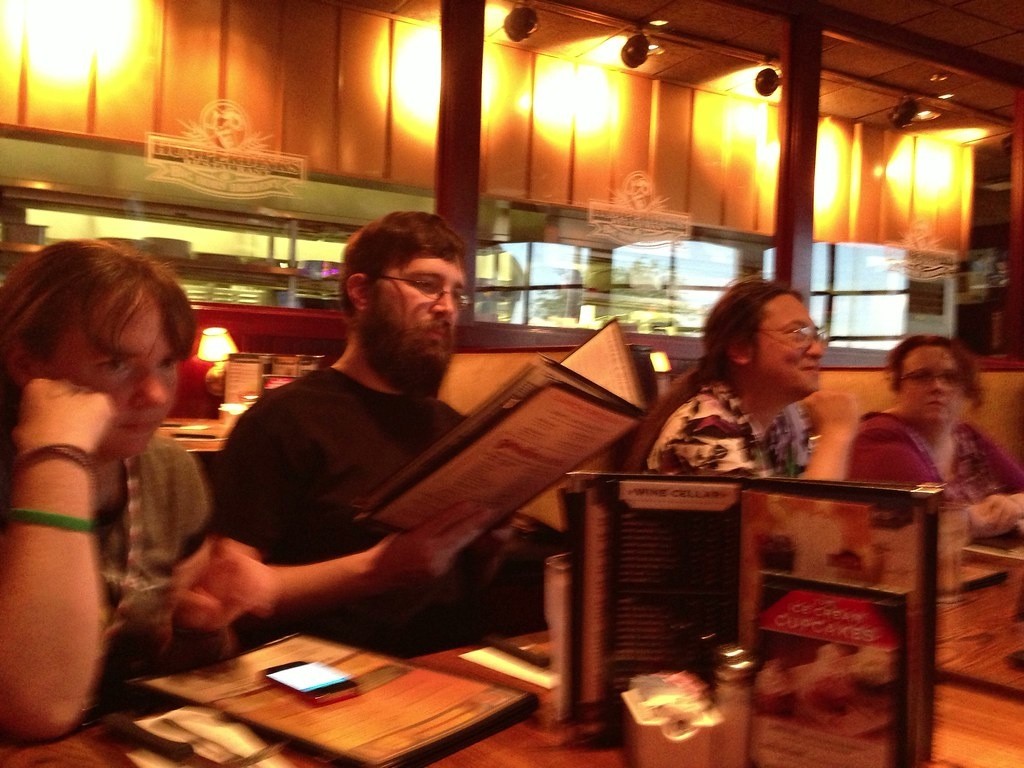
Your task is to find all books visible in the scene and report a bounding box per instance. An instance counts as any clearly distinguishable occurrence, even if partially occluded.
[963,566,1009,592]
[136,631,542,768]
[342,315,649,554]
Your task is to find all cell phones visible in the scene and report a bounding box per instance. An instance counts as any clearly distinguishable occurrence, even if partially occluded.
[260,661,358,704]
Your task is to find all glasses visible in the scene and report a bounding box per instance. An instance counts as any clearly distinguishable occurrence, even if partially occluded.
[896,371,963,386]
[756,327,823,345]
[372,274,472,311]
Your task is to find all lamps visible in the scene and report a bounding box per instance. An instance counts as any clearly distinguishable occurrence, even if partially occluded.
[887,92,917,129]
[197,326,238,396]
[755,53,781,97]
[502,0,541,41]
[621,19,650,69]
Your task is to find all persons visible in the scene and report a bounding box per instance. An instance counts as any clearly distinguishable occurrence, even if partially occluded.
[0,239,236,745]
[174,211,581,660]
[476,243,524,324]
[843,333,1024,540]
[619,275,826,644]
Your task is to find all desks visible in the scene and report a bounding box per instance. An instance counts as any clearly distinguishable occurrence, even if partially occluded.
[157,418,228,463]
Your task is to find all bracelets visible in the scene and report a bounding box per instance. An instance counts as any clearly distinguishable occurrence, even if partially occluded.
[4,507,98,533]
[10,441,100,483]
